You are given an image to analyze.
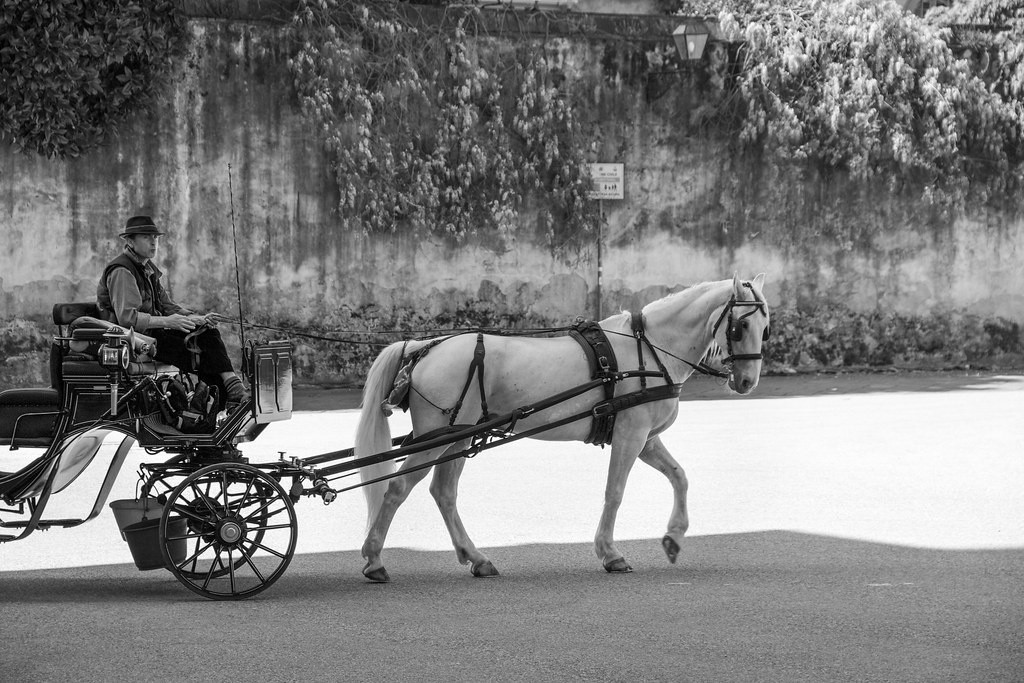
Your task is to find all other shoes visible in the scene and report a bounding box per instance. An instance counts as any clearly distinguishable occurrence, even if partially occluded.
[225,388,252,408]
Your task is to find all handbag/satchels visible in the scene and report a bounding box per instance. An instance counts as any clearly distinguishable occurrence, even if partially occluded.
[162,379,219,434]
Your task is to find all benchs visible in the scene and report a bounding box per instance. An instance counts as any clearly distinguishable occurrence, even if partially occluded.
[60,304,180,380]
[0,304,125,439]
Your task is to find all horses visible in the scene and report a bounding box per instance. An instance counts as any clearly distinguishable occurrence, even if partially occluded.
[354,271,768,584]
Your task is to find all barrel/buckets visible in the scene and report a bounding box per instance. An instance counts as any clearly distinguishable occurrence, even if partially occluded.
[109,498,165,541]
[121,517,189,571]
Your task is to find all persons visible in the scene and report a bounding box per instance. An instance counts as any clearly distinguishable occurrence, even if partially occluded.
[96,215,249,413]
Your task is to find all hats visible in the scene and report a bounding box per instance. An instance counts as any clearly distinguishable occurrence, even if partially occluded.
[119,216,165,238]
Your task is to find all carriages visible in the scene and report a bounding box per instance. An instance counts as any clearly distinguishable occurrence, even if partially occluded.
[1,215,774,602]
